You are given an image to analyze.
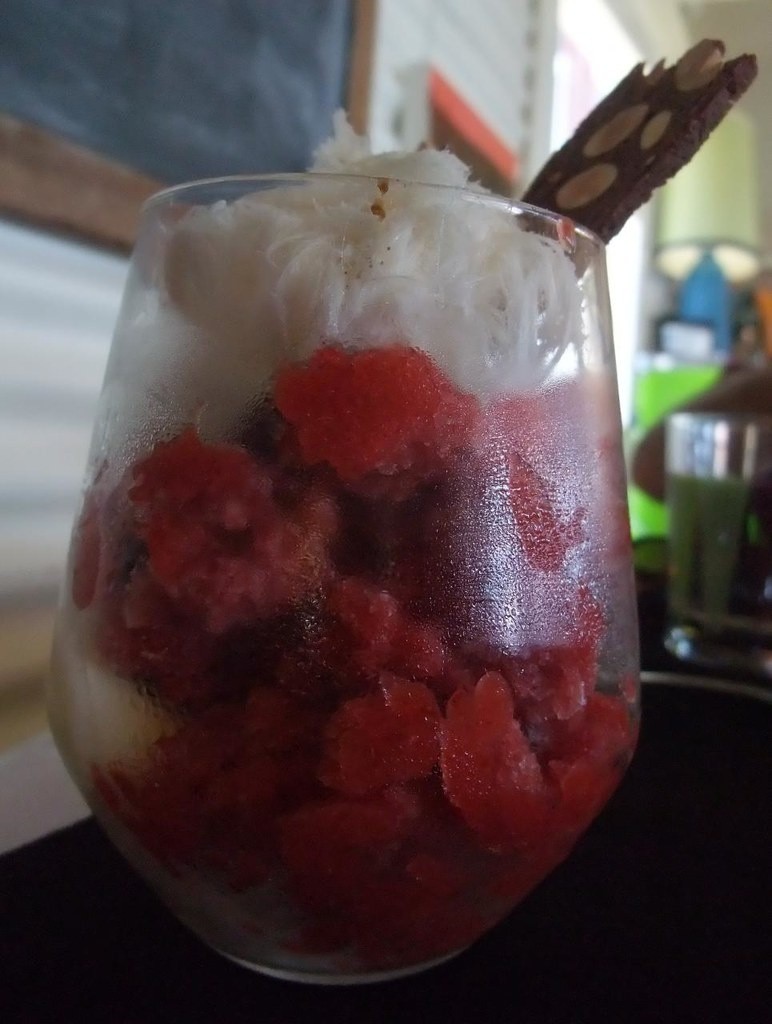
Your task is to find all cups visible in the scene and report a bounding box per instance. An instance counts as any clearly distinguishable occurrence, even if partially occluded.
[658,411,772,686]
[45,171,642,984]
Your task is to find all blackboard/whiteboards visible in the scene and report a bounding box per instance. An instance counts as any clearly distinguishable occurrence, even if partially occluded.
[0,1,379,276]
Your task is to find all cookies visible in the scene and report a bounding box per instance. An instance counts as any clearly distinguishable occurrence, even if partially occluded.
[517,37,759,285]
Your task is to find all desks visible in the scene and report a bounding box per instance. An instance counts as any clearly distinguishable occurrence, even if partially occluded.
[0,536,772,1024]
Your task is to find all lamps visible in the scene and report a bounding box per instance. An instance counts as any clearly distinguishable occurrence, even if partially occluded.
[653,107,757,342]
